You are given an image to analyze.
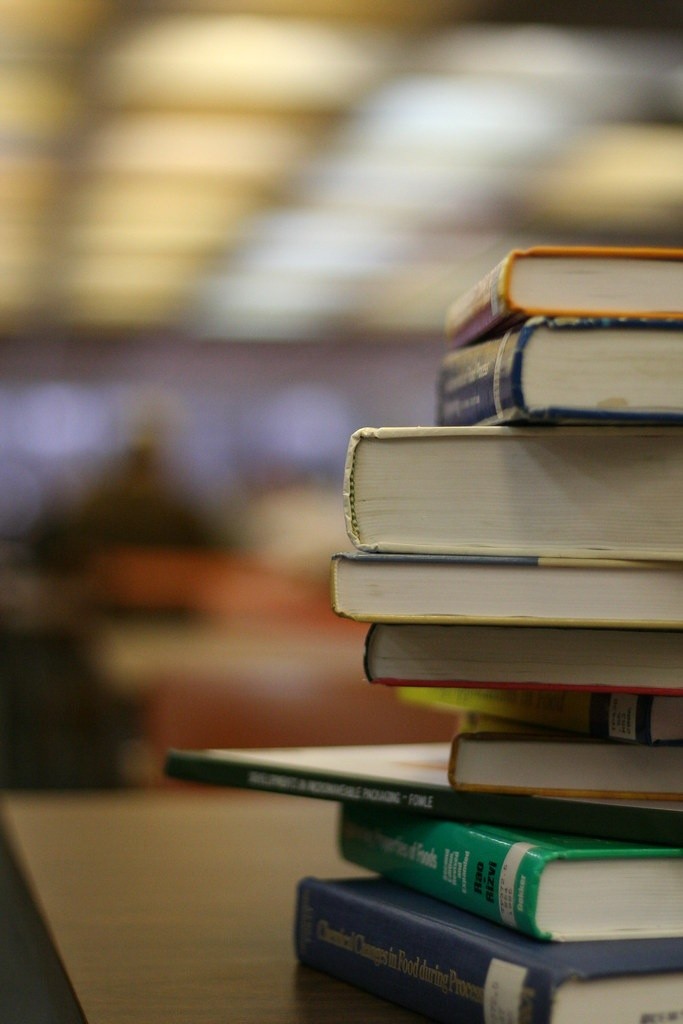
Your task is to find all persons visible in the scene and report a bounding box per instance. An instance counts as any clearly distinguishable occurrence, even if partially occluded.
[37,440,348,790]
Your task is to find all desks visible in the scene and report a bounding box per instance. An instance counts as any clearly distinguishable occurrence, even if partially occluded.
[0,784,483,1024]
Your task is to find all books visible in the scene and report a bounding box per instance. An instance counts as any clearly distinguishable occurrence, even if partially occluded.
[332,240,683,798]
[162,741,682,1024]
